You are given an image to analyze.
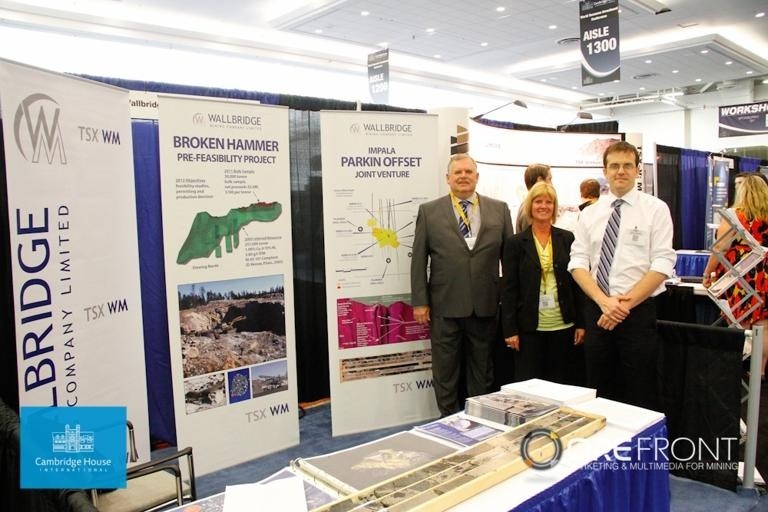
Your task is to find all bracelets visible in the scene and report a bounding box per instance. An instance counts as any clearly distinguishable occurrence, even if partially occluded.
[703,271,712,280]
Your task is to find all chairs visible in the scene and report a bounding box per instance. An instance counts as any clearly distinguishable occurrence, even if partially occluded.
[54,420,196,512]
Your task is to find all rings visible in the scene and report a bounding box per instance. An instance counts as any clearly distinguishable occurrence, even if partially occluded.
[506,344,512,349]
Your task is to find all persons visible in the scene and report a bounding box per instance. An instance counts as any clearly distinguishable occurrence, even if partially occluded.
[568,141,678,415]
[499,180,587,386]
[412,154,515,415]
[578,178,598,220]
[734,172,750,196]
[516,163,552,234]
[702,175,768,382]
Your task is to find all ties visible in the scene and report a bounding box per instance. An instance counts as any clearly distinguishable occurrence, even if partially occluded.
[596,201,622,297]
[457,201,474,238]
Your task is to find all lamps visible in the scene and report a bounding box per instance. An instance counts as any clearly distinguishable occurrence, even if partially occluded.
[558,112,592,133]
[472,101,527,121]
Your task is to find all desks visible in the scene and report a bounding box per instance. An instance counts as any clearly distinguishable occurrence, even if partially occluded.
[676,249,712,275]
[165,397,669,512]
